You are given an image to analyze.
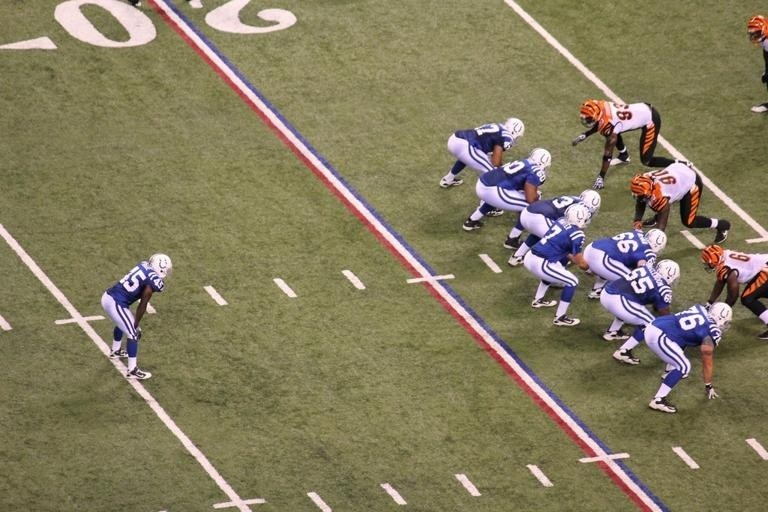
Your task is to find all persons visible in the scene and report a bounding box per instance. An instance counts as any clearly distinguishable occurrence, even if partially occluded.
[523,203,592,326]
[100,254,173,382]
[644,301,733,413]
[505,190,601,267]
[572,99,693,194]
[701,244,768,340]
[747,15,768,114]
[583,227,668,301]
[599,258,680,366]
[630,162,732,245]
[462,148,552,251]
[439,118,525,218]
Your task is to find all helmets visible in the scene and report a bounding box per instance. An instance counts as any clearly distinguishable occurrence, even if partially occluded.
[644,226,668,258]
[710,301,732,333]
[576,100,604,129]
[627,174,652,201]
[654,258,681,290]
[502,117,526,144]
[565,203,592,230]
[746,13,768,48]
[701,243,724,274]
[149,253,172,283]
[529,148,554,174]
[580,188,601,216]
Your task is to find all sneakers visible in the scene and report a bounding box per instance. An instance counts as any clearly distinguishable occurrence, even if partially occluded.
[531,297,558,308]
[508,252,525,267]
[679,159,694,170]
[714,219,731,245]
[613,348,640,365]
[756,330,768,340]
[648,396,678,413]
[587,287,603,299]
[610,152,631,166]
[487,209,505,217]
[440,176,465,188]
[551,313,581,328]
[503,234,523,250]
[602,328,630,341]
[126,366,152,380]
[110,349,128,359]
[462,218,482,231]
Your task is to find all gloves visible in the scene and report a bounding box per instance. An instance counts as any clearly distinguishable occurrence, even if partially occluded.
[593,171,605,190]
[702,300,712,311]
[572,134,586,147]
[704,383,719,400]
[135,327,143,340]
